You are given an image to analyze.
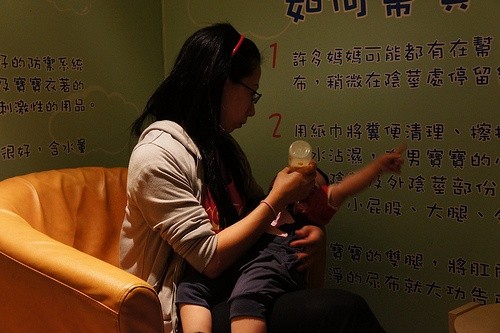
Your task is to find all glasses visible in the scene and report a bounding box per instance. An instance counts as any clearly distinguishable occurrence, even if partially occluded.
[233,78,263,105]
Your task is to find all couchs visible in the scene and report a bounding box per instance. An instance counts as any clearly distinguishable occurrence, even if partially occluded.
[0,168,165,333]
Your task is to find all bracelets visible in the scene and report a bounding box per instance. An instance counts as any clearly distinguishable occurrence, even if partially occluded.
[259,200,278,217]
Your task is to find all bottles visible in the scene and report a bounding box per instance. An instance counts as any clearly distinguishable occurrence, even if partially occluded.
[286,139,313,169]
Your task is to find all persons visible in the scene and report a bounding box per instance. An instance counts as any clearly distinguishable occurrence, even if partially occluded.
[120,22,387,333]
[176,142,408,333]
